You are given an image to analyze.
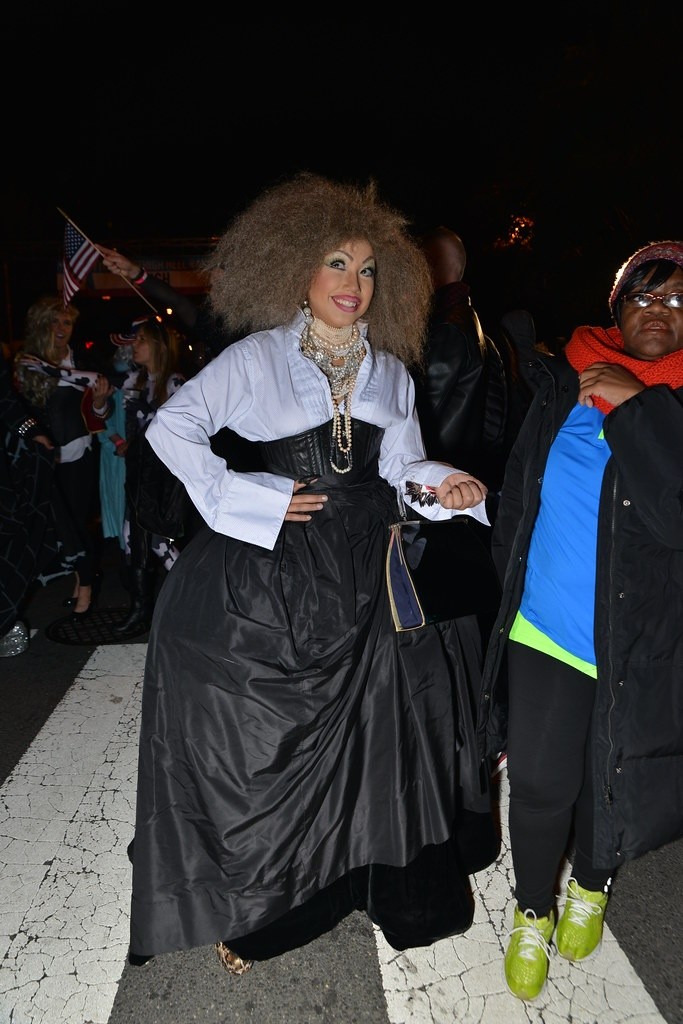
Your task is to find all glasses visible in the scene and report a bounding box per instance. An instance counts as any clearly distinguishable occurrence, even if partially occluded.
[620,292,683,309]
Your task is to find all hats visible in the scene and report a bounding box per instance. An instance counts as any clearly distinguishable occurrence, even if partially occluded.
[608,239,683,330]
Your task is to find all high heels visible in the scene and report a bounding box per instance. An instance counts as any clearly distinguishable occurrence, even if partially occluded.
[64,596,78,607]
[71,583,97,622]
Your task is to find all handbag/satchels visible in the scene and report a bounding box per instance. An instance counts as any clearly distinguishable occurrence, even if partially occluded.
[386,461,504,633]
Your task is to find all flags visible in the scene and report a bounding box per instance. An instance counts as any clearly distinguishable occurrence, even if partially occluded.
[63,220,100,311]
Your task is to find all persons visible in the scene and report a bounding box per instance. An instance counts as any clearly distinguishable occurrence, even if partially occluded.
[0,245,243,636]
[413,223,564,770]
[130,182,487,975]
[478,242,683,1001]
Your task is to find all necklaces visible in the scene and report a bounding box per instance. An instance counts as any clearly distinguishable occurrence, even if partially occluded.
[302,324,366,474]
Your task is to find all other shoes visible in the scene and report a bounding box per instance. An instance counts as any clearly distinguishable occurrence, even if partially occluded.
[214,941,253,975]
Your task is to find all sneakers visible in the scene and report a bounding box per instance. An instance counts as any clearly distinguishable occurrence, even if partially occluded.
[502,902,558,1000]
[555,877,609,962]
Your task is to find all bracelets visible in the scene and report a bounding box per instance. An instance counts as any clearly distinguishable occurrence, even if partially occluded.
[406,481,439,507]
[132,268,147,285]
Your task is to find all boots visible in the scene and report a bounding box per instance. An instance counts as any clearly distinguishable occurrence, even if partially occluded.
[111,567,153,632]
